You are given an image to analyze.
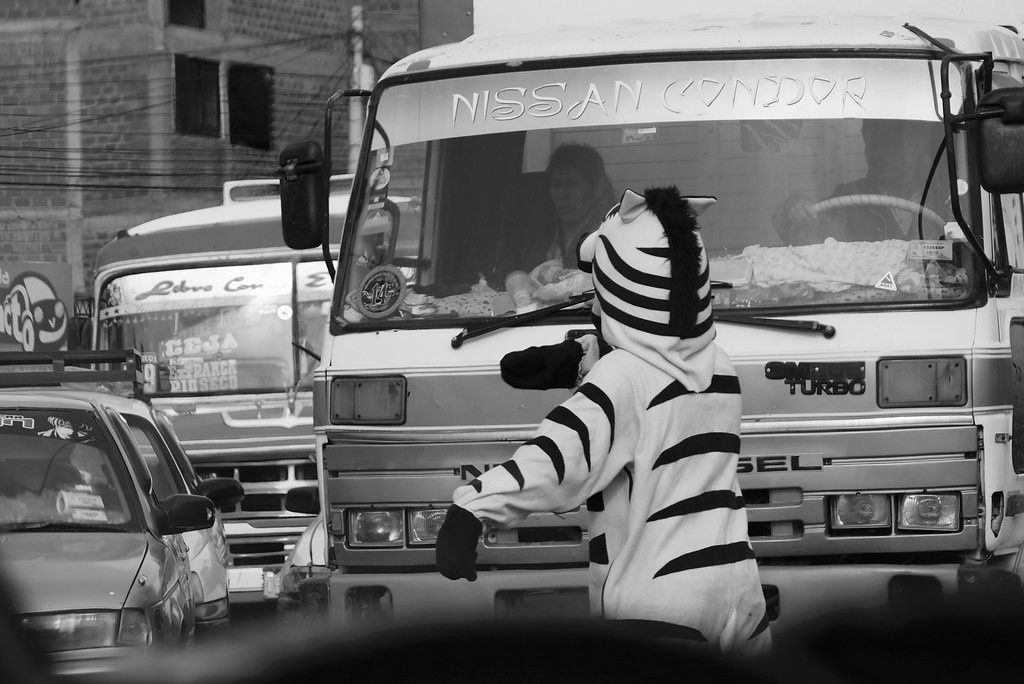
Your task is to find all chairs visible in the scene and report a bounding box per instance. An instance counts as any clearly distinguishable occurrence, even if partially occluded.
[495,173,615,287]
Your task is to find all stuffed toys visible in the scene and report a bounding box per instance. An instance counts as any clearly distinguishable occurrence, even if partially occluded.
[436,186,773,663]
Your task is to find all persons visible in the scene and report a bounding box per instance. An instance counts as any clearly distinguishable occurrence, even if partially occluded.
[774,118,965,247]
[524,144,625,275]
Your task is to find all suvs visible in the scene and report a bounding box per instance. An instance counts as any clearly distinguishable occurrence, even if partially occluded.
[1,348,243,684]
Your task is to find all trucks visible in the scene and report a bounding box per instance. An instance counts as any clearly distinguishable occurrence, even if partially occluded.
[276,17,1024,649]
[61,175,425,603]
